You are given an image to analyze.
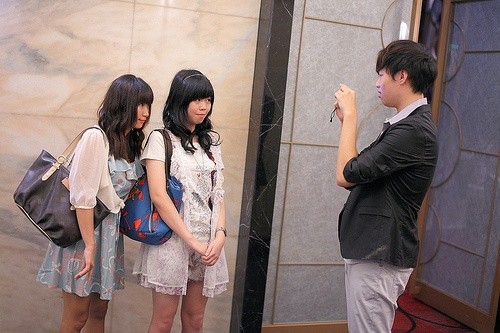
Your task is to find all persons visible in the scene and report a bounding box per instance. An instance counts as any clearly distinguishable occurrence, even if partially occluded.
[120,68,227,333]
[14,74,154,333]
[330,39,440,333]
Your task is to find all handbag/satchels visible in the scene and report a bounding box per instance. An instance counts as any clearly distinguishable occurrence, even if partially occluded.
[13,126,125,248]
[119,129,183,245]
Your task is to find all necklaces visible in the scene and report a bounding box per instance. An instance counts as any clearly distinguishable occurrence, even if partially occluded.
[183,141,204,174]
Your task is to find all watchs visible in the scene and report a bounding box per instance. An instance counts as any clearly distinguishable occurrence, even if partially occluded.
[215,226,227,237]
